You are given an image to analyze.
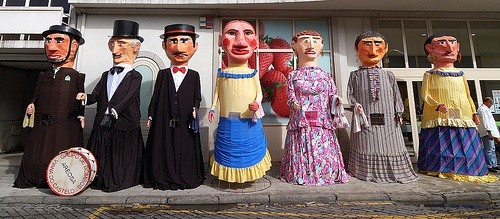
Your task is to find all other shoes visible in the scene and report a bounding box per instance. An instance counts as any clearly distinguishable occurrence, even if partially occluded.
[488,168,497,172]
[492,166,500,170]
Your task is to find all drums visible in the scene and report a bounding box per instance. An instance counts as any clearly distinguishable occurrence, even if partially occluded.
[45,147,97,197]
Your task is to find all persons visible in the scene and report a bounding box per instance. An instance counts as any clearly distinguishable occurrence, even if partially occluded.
[14,24,85,188]
[478,97,499,172]
[275,29,350,184]
[75,20,146,192]
[208,20,272,183]
[142,23,206,190]
[346,31,420,182]
[417,32,499,183]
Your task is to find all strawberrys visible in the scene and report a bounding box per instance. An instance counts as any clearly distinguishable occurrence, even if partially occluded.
[222,20,294,118]
